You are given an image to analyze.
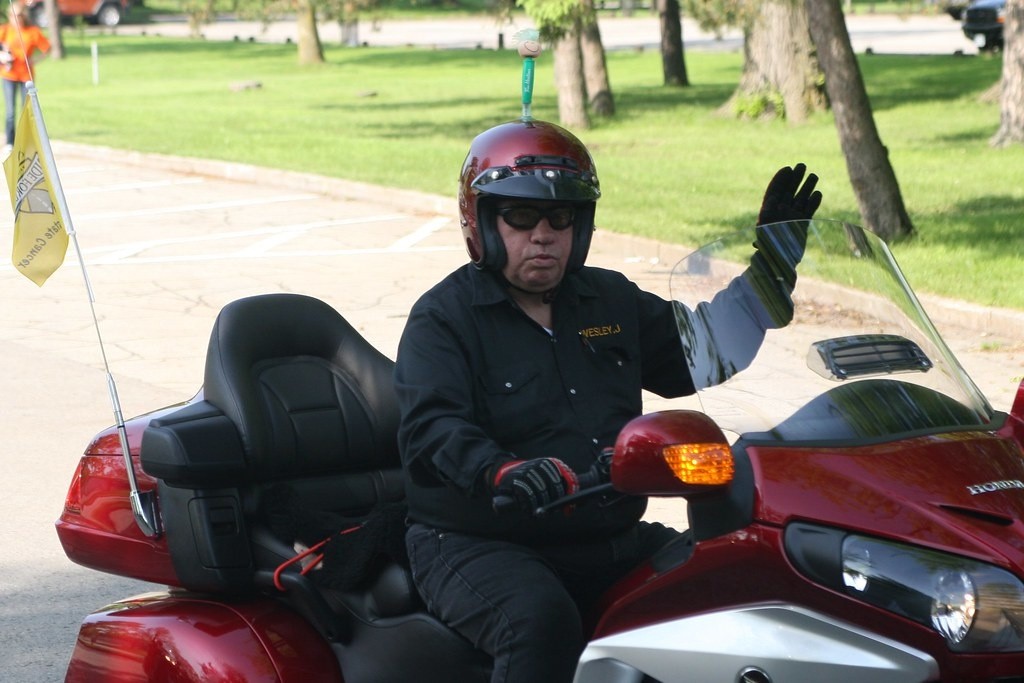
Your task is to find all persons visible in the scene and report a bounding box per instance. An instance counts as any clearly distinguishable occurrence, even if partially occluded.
[392,118,824,683]
[0,4,50,149]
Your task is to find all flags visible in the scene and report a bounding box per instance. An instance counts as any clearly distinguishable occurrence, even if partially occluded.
[3,93,68,288]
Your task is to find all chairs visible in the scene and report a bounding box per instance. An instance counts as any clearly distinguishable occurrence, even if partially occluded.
[138,292,492,683]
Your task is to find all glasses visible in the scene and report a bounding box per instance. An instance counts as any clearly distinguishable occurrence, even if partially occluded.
[494,201,576,231]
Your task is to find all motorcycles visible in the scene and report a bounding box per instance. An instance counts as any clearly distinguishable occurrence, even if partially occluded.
[56,215,1023,683]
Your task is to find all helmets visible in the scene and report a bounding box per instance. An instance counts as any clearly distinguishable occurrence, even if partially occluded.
[458,119,601,275]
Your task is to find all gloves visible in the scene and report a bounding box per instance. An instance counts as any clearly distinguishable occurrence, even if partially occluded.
[751,162,822,293]
[488,454,580,529]
